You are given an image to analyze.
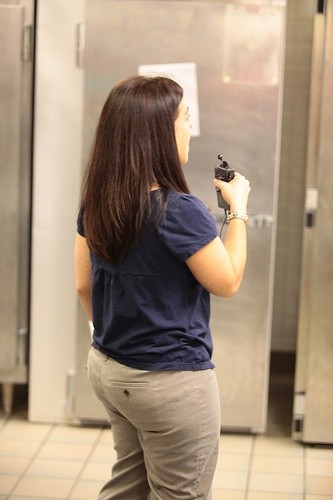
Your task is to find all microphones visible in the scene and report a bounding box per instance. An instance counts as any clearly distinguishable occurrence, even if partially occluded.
[218,154,228,167]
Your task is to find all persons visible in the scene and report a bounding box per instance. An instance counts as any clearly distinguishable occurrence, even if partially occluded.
[73,74,250,500]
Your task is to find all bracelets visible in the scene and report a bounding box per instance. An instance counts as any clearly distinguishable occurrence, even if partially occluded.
[227,212,249,222]
